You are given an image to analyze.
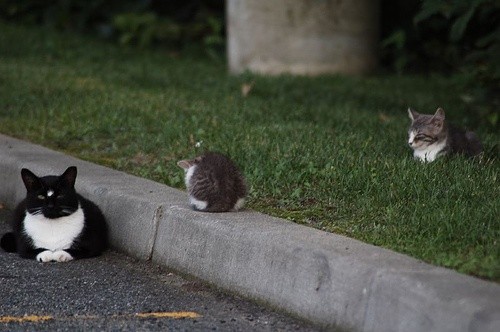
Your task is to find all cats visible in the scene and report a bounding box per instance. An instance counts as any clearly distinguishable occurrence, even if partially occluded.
[408,106,481,163]
[177,151,248,213]
[0,166,109,264]
